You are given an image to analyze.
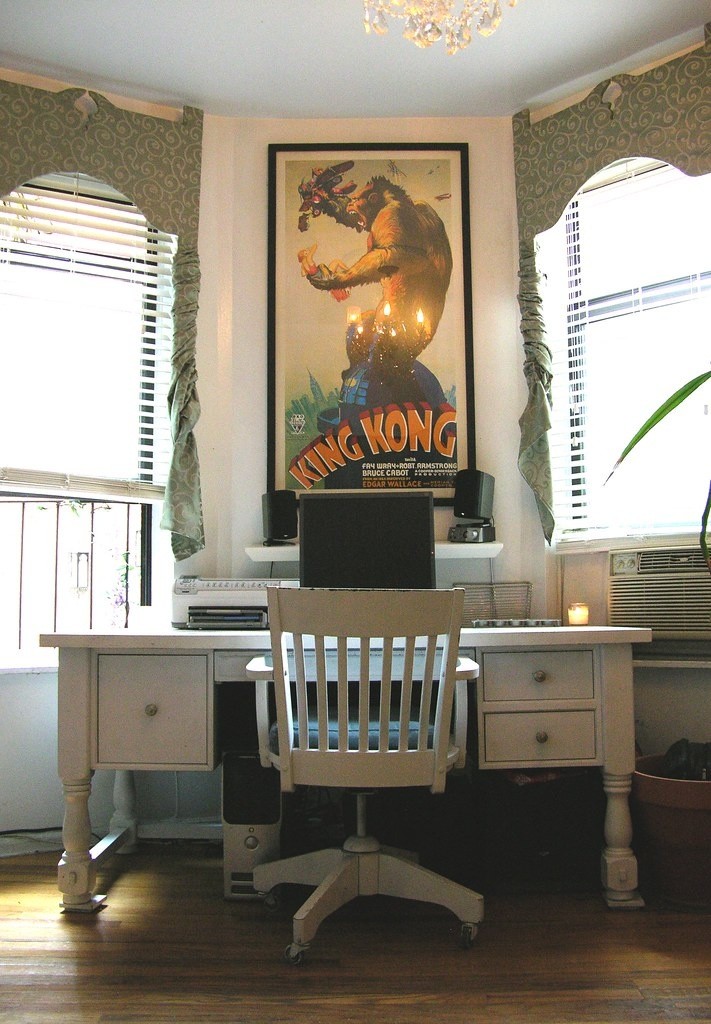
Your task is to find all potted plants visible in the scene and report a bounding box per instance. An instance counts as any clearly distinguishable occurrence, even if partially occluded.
[627,738,711,907]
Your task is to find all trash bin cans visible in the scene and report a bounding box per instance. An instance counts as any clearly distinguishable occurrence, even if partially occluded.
[630,735,710,911]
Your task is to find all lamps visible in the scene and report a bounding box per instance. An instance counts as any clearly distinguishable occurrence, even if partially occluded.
[363,1,517,57]
[567,602,589,626]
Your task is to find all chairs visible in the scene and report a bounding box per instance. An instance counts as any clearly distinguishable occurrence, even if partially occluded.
[246,587,486,965]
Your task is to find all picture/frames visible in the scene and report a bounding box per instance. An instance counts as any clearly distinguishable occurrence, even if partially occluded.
[266,142,475,506]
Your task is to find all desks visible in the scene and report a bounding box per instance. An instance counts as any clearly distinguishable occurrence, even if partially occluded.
[39,626,651,916]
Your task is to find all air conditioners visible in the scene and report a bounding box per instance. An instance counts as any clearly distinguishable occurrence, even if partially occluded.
[606,545,711,669]
